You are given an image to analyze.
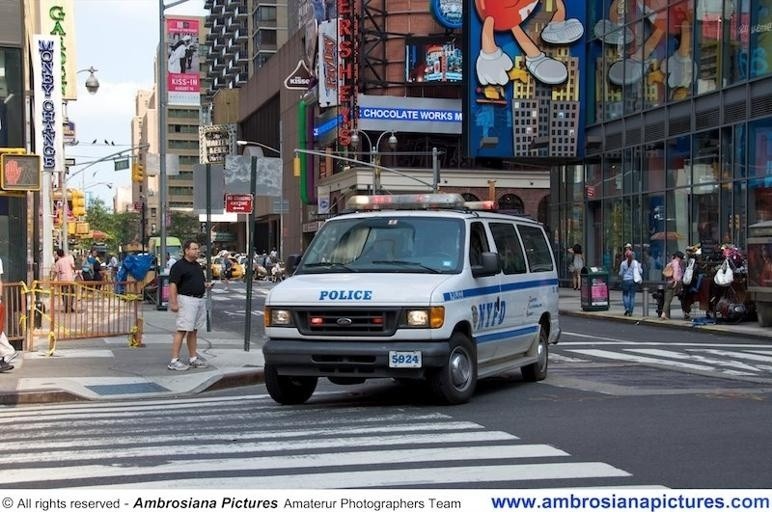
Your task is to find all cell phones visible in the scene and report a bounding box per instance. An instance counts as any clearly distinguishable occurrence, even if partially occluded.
[211,283,215,286]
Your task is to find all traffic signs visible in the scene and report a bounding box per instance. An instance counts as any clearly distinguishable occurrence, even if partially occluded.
[223,194,254,214]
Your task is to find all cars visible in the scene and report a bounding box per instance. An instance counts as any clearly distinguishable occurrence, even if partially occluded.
[603,217,717,282]
[90,235,287,283]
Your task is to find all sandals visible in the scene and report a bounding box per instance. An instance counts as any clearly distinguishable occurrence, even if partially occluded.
[661,315,670,320]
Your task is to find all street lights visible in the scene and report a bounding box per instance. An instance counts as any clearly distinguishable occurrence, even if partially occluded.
[61,143,150,274]
[236,140,285,264]
[348,130,398,197]
[61,68,101,255]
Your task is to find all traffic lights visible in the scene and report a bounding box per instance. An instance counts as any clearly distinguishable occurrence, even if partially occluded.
[132,163,145,184]
[1,153,43,192]
[729,213,740,230]
[73,190,85,217]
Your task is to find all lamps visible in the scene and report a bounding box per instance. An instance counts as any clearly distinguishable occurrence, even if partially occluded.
[76,65,99,93]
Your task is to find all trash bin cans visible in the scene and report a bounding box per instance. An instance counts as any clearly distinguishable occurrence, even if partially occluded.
[156,275,170,311]
[579,266,610,311]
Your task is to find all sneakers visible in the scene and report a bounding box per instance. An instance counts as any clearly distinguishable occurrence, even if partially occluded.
[188,356,209,368]
[624,307,629,316]
[167,357,191,371]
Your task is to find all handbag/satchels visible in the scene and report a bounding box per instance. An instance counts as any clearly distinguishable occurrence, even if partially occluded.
[633,260,643,285]
[567,263,574,273]
[681,238,750,324]
[662,263,673,277]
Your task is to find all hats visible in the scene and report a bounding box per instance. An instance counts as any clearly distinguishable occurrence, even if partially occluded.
[672,250,685,258]
[624,243,632,248]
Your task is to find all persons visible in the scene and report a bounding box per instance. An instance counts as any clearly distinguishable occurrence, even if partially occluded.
[165,251,178,276]
[660,246,694,322]
[568,243,586,292]
[0,252,18,376]
[166,238,214,372]
[166,31,199,75]
[615,249,644,318]
[623,242,637,263]
[81,249,118,294]
[237,246,278,284]
[220,250,235,292]
[49,249,77,314]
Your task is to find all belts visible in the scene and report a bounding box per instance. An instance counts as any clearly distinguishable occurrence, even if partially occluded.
[182,294,203,298]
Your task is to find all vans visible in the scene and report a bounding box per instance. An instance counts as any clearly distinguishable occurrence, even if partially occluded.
[263,192,562,405]
